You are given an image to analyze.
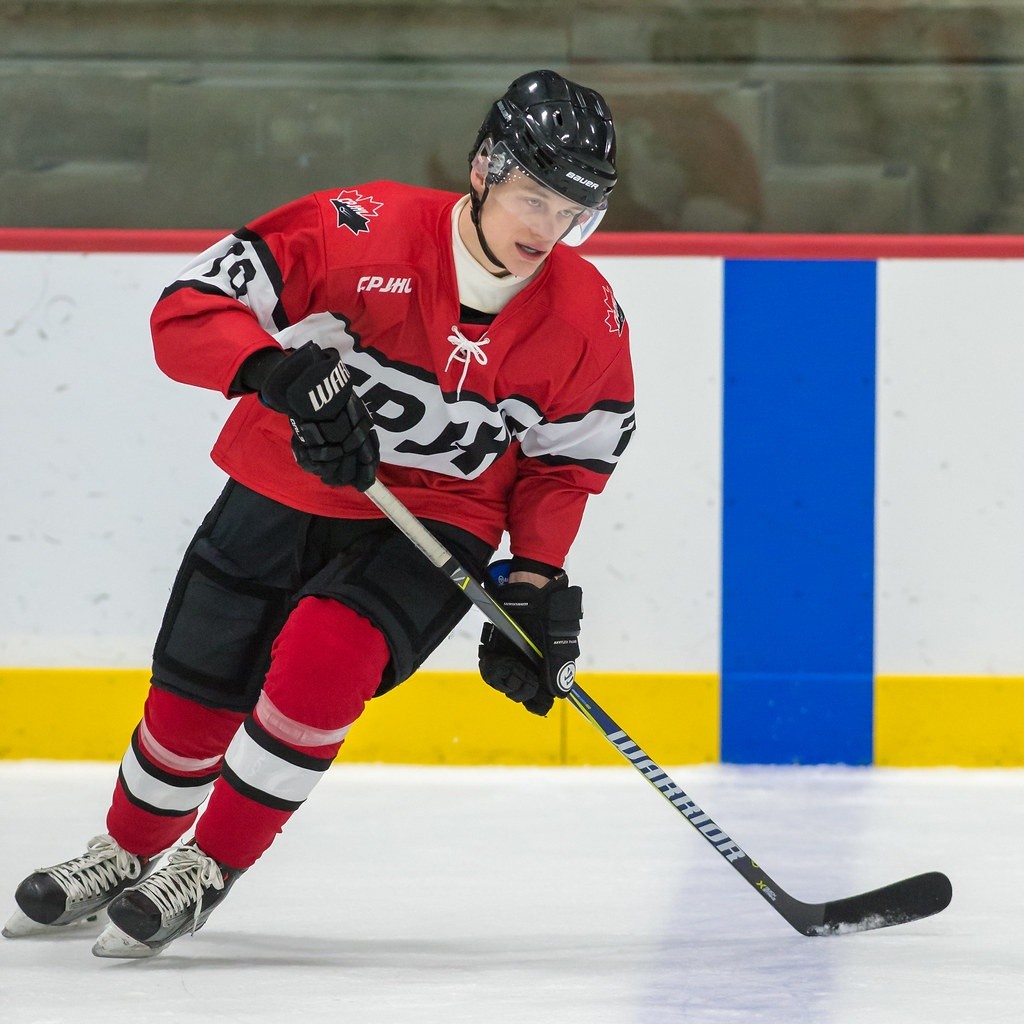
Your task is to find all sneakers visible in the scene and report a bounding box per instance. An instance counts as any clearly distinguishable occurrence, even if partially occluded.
[0,833,168,938]
[92,836,246,958]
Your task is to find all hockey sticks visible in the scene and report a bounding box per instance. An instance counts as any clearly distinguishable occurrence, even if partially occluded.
[364,477,954,938]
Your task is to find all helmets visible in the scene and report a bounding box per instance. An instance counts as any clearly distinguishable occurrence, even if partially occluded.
[490,69,617,209]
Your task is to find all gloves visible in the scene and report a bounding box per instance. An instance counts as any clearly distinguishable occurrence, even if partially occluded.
[257,340,380,492]
[478,560,580,716]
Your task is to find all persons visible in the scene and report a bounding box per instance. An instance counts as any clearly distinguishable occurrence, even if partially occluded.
[2,70,636,975]
